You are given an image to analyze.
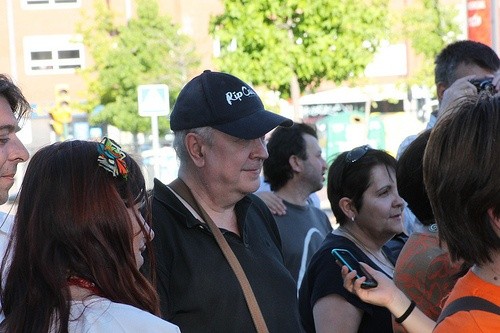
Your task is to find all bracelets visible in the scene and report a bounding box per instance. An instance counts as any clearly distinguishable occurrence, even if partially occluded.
[394,300,415,323]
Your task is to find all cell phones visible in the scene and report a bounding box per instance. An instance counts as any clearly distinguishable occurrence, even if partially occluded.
[332,249,378,289]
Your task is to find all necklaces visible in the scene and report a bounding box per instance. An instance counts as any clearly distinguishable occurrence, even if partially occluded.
[338,227,395,271]
[65,277,101,296]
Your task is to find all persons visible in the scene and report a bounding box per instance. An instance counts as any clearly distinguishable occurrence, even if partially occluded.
[0,73,29,324]
[141,69,306,333]
[265,121,334,301]
[299,146,405,333]
[0,137,181,333]
[253,40,500,333]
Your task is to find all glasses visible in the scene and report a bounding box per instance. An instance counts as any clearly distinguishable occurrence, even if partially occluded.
[340,144,371,184]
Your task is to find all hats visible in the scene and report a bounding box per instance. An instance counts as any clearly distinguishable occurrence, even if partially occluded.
[170,70,294,139]
